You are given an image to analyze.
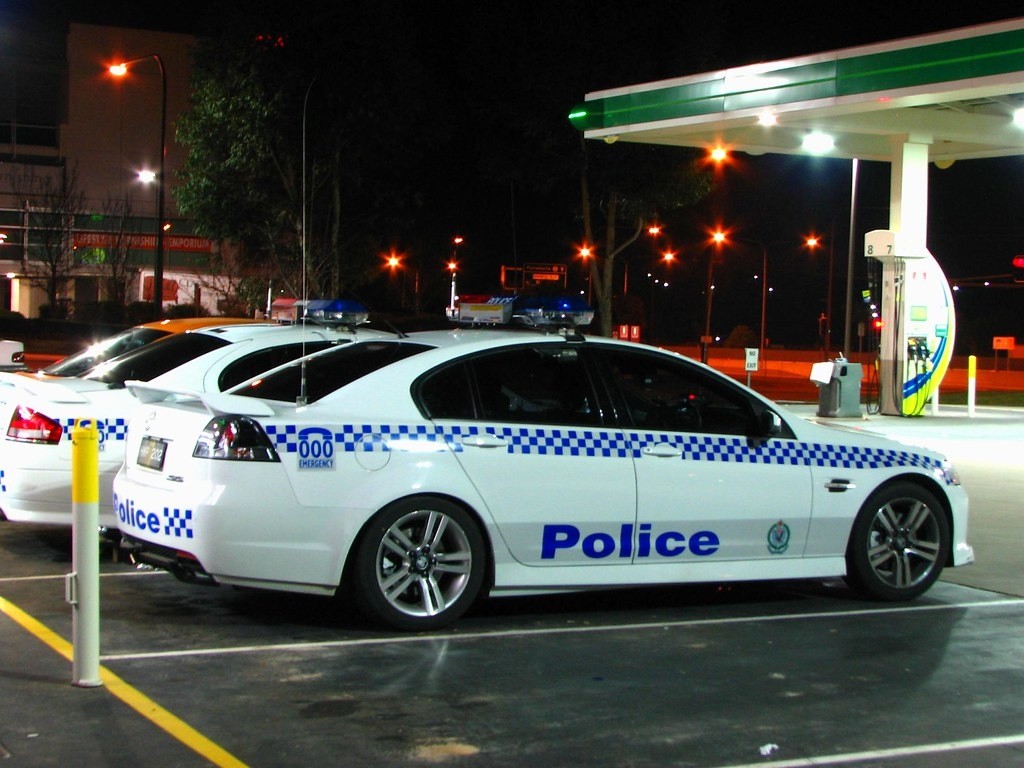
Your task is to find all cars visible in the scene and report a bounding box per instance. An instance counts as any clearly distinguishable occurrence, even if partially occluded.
[0,296,486,532]
[106,294,977,634]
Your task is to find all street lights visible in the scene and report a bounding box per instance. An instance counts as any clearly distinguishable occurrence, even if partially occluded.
[107,53,169,321]
[449,236,464,318]
[700,231,725,364]
